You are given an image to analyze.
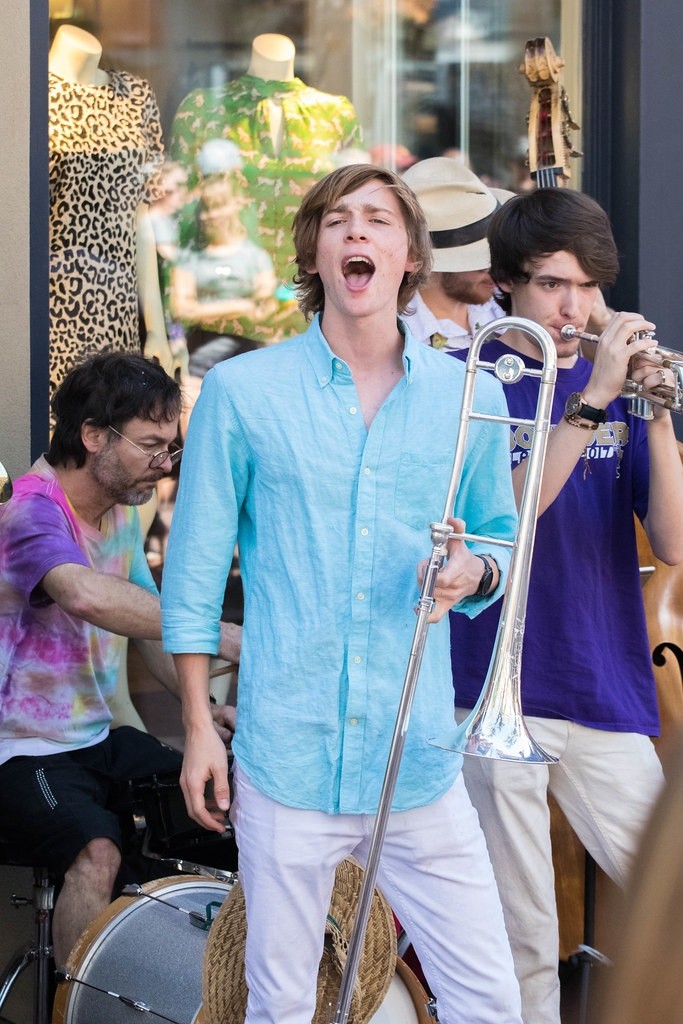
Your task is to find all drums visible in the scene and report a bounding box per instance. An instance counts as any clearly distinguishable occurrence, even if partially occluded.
[54,874,438,1024]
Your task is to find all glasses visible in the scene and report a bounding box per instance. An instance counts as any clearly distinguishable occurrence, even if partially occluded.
[108,426,183,471]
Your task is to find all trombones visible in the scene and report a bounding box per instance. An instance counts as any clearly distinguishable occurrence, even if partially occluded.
[333,315,559,1023]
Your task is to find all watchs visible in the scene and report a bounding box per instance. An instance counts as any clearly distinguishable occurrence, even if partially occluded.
[565,391,609,424]
[475,555,494,599]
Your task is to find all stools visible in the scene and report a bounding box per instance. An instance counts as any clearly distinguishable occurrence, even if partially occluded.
[0,839,60,1024]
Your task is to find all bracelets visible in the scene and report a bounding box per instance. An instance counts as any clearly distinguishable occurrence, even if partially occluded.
[564,415,599,431]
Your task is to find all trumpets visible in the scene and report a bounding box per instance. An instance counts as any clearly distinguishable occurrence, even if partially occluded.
[561,324,683,419]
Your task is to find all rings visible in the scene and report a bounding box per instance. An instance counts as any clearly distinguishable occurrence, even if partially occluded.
[657,370,666,383]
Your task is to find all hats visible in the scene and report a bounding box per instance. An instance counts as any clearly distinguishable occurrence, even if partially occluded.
[201,858,398,1024]
[401,156,517,275]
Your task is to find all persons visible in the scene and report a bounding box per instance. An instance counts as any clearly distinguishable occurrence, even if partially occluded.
[0,352,242,974]
[402,156,683,1024]
[162,164,523,1024]
[39,25,172,439]
[171,32,371,394]
